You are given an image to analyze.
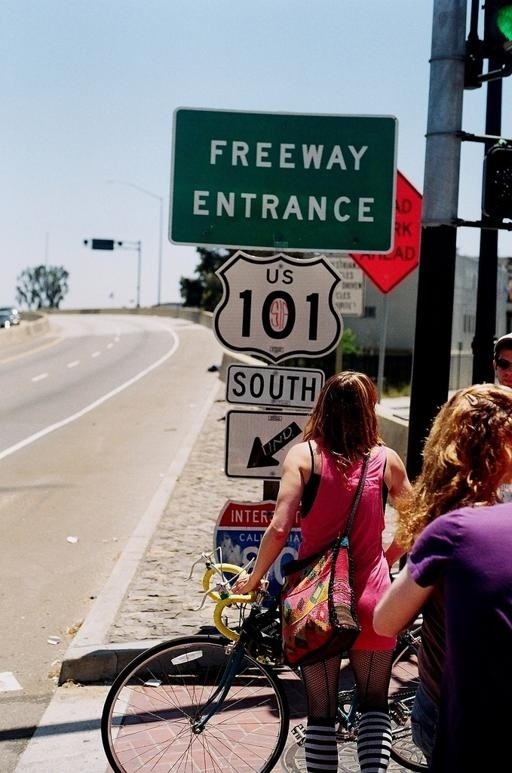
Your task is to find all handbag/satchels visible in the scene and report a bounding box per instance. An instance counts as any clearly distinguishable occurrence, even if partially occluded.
[280,535,360,667]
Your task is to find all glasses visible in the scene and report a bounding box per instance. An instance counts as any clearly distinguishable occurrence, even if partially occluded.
[497,359,512,369]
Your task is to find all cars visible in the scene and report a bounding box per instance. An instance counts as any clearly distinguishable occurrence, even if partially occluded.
[0,306,24,328]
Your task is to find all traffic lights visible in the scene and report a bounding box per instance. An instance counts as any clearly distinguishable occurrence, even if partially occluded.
[480,1,512,65]
[475,139,511,218]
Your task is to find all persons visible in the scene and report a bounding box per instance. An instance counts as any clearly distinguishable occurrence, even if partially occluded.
[232,371,415,773]
[492,332,512,389]
[373,382,512,773]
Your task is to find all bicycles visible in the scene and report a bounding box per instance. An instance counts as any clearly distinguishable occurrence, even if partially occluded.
[96,551,446,773]
